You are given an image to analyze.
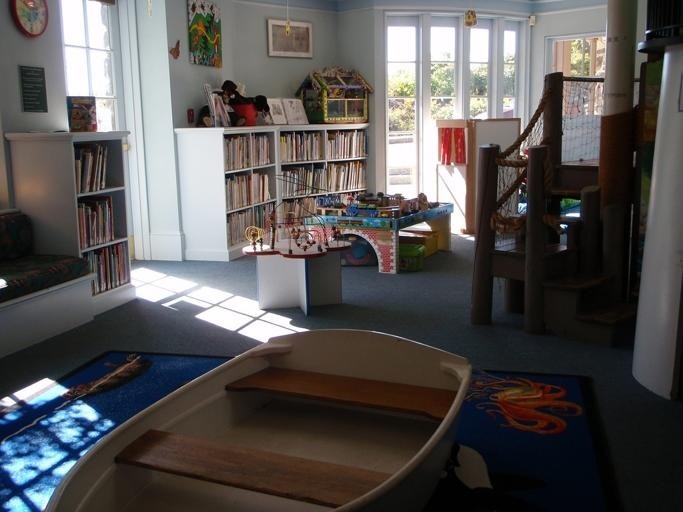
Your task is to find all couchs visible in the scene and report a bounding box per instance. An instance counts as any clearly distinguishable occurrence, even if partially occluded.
[0,207,98,361]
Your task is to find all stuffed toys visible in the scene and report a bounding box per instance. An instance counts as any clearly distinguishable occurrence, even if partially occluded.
[222,80,270,126]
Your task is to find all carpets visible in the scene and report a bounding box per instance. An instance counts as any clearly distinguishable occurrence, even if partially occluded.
[0,348,623,512]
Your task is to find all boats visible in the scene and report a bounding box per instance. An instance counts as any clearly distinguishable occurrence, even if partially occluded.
[44,328,472,512]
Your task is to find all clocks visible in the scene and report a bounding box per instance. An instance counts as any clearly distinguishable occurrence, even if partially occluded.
[10,0,49,39]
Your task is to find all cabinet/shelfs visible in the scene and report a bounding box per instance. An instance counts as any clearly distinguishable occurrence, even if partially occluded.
[2,129,138,317]
[173,122,372,263]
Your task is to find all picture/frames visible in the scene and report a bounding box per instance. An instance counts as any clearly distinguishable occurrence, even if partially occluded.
[282,98,309,125]
[266,97,287,125]
[267,19,313,58]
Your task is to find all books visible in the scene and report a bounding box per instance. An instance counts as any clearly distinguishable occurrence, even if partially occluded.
[224,129,368,246]
[74,143,131,295]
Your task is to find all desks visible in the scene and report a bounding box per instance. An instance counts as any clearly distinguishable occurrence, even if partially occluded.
[241,224,353,319]
[303,202,455,275]
